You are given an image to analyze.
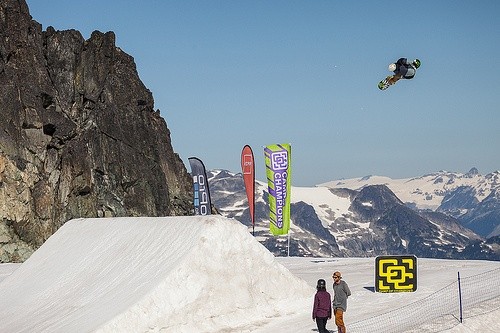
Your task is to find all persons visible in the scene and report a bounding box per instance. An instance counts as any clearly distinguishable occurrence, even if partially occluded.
[312,279,331,333]
[387,58,418,80]
[332,271,351,333]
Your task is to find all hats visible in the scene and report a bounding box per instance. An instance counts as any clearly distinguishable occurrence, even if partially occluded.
[334,272,342,278]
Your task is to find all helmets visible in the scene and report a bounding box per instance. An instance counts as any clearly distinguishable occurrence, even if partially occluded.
[388,62,396,71]
[317,279,325,287]
[412,59,421,68]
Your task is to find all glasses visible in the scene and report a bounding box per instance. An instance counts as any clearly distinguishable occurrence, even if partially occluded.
[333,276,338,278]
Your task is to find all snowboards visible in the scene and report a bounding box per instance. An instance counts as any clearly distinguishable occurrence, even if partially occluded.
[377,58,421,91]
